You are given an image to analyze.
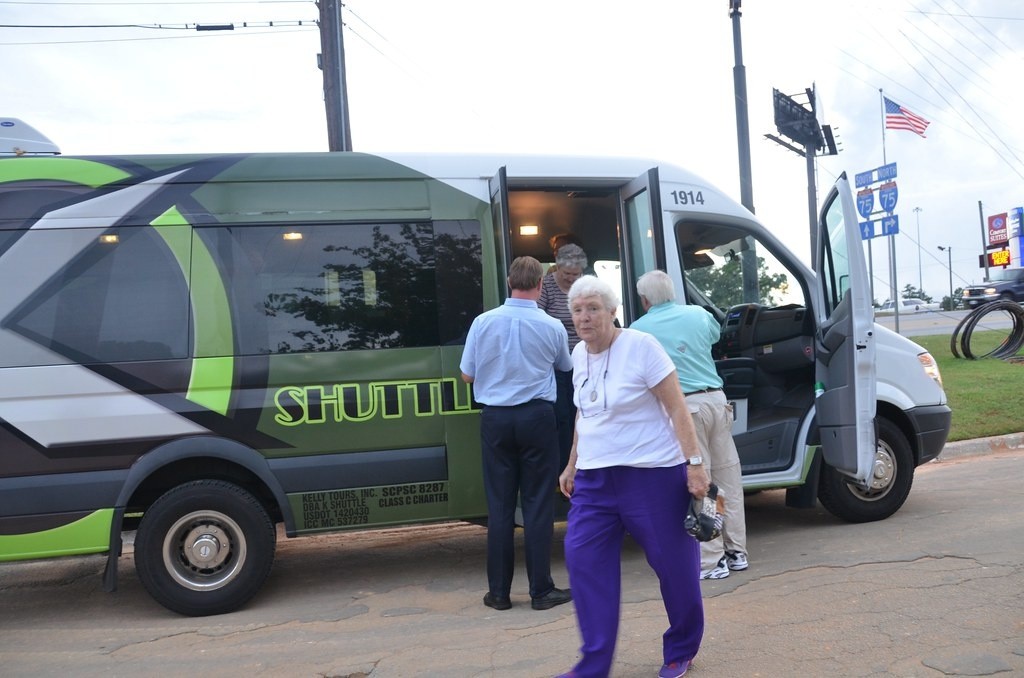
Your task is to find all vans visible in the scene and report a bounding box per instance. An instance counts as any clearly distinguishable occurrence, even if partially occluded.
[0,116,954,617]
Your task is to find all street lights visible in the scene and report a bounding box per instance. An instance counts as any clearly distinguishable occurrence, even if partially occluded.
[937,246,954,311]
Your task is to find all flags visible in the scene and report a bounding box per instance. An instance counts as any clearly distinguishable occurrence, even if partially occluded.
[883,96,930,139]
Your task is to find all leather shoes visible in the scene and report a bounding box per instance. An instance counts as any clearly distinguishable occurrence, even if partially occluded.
[483,591,512,609]
[532,588,572,611]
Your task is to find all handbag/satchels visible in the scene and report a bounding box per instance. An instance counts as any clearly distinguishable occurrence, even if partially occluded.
[683,481,725,543]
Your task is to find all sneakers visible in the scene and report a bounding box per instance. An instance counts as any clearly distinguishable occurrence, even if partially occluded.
[553,671,575,678]
[658,658,692,678]
[699,557,730,579]
[726,550,748,570]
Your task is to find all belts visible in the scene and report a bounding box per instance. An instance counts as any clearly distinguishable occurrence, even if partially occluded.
[684,387,723,396]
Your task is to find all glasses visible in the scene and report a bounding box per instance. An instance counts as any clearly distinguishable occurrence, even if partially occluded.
[579,370,608,419]
[557,266,581,279]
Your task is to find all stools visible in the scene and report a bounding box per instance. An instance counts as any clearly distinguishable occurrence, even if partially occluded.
[714,356,756,398]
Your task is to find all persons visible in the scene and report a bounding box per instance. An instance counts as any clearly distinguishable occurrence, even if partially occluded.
[546,275,709,678]
[540,235,595,464]
[459,256,573,610]
[628,270,749,580]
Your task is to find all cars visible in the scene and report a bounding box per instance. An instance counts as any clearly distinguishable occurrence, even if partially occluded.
[875,298,945,313]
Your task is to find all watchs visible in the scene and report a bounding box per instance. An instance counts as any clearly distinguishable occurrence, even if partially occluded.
[684,456,705,467]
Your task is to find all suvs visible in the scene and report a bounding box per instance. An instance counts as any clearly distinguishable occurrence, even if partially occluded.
[962,267,1024,308]
